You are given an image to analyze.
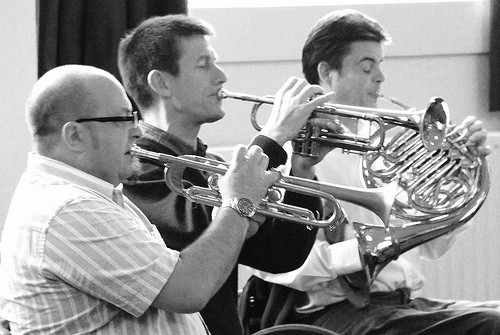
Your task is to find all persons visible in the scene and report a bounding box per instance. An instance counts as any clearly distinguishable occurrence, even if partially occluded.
[117,13,344,335]
[249,10,500,335]
[0,64,286,335]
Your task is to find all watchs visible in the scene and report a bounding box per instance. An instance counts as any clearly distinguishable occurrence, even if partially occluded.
[221,197,256,219]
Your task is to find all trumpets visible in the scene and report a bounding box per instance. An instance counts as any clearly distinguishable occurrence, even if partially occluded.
[128,143,400,232]
[215,84,450,158]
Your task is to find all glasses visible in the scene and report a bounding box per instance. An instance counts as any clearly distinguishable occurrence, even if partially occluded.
[74,111,139,128]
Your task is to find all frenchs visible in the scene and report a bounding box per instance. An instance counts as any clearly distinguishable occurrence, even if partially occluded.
[352,92,491,289]
